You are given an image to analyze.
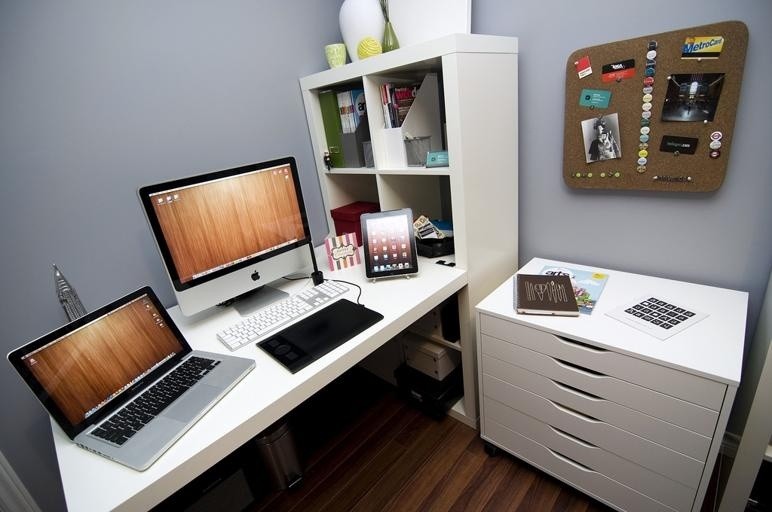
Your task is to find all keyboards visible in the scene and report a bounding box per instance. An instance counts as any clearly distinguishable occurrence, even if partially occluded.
[217,278,351,353]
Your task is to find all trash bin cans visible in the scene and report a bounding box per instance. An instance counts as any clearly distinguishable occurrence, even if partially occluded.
[255,424,309,497]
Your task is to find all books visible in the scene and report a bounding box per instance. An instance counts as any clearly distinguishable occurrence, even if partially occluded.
[515,273,580,318]
[336,81,421,134]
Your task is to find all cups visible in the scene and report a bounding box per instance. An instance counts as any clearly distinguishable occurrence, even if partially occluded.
[325,43,347,69]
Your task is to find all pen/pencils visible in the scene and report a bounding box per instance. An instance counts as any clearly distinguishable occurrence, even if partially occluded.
[405,131,413,139]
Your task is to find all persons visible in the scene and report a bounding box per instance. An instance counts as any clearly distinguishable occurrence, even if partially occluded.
[593,120,616,161]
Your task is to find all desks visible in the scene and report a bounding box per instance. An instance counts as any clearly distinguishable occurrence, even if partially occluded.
[48,244,465,512]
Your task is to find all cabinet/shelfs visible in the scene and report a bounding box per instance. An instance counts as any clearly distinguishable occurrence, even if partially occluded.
[474,257,748,510]
[300,36,518,428]
[717,277,771,511]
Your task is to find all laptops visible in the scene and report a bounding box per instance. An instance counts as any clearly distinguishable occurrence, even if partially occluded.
[6,286,255,471]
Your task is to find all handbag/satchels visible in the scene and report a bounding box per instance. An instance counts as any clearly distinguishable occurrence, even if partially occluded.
[325,231,362,272]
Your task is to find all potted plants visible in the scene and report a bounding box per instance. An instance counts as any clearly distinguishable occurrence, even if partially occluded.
[379,0,400,53]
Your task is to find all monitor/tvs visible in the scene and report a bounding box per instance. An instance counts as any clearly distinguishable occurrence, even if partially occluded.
[137,156,315,317]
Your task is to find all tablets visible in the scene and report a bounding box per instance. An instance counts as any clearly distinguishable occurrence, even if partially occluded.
[361,208,418,280]
